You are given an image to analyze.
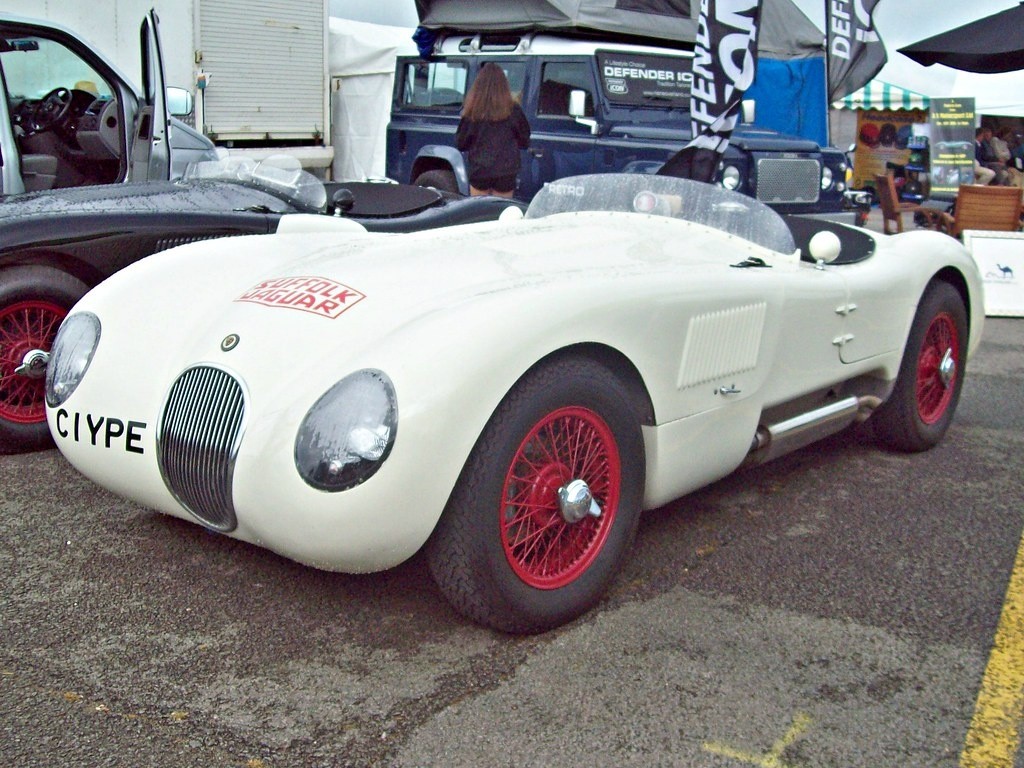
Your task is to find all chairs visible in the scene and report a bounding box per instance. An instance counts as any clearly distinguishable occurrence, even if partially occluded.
[942,182,1024,238]
[872,170,945,234]
[21,152,57,191]
[431,88,463,106]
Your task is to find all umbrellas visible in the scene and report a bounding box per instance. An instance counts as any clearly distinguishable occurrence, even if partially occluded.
[896,1,1024,73]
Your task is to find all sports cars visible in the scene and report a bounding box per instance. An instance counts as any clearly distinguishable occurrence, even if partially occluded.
[40,138,990,642]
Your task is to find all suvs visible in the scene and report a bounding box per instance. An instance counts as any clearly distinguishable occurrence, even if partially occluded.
[379,1,879,233]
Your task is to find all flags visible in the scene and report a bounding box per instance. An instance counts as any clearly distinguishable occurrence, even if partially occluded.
[654,1,764,189]
[826,0,887,105]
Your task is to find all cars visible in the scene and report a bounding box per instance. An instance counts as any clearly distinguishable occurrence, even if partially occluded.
[0,14,520,459]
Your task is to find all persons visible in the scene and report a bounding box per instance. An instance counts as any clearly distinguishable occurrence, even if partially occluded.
[974,127,1024,186]
[454,63,530,198]
